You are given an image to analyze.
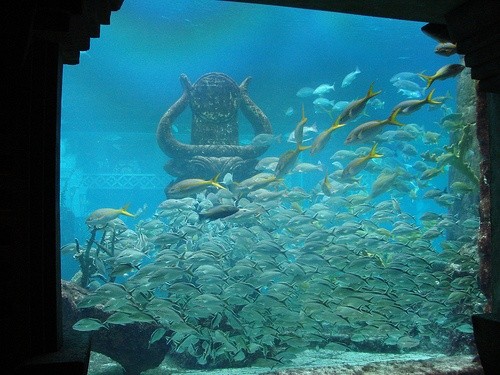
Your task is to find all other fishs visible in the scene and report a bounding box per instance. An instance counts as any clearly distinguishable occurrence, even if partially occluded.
[61,40,490,367]
